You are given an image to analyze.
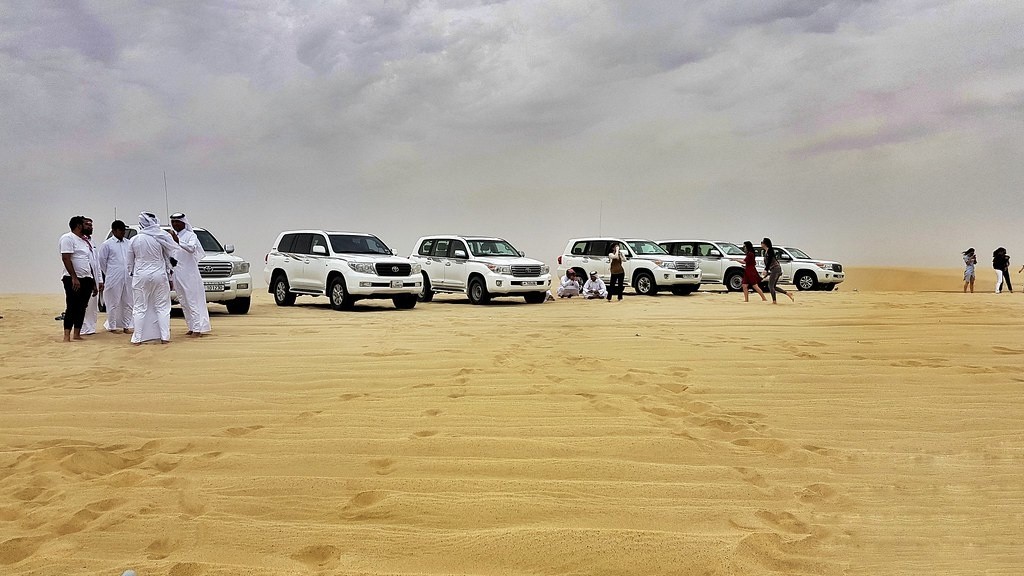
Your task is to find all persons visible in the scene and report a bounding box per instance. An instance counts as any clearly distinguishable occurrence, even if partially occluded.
[56,209,213,346]
[992,247,1024,294]
[582,270,608,299]
[556,268,579,299]
[729,241,768,302]
[605,241,627,302]
[544,290,555,303]
[760,237,795,304]
[961,247,977,293]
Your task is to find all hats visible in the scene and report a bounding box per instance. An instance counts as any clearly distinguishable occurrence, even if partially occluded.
[113,220,129,229]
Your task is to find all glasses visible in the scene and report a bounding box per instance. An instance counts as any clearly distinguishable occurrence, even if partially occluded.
[743,246,746,247]
[761,242,765,244]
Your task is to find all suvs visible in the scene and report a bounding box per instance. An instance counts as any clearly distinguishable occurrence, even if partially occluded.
[263,228,426,311]
[99,225,253,315]
[406,233,554,304]
[732,243,846,292]
[656,242,783,290]
[557,241,705,296]
